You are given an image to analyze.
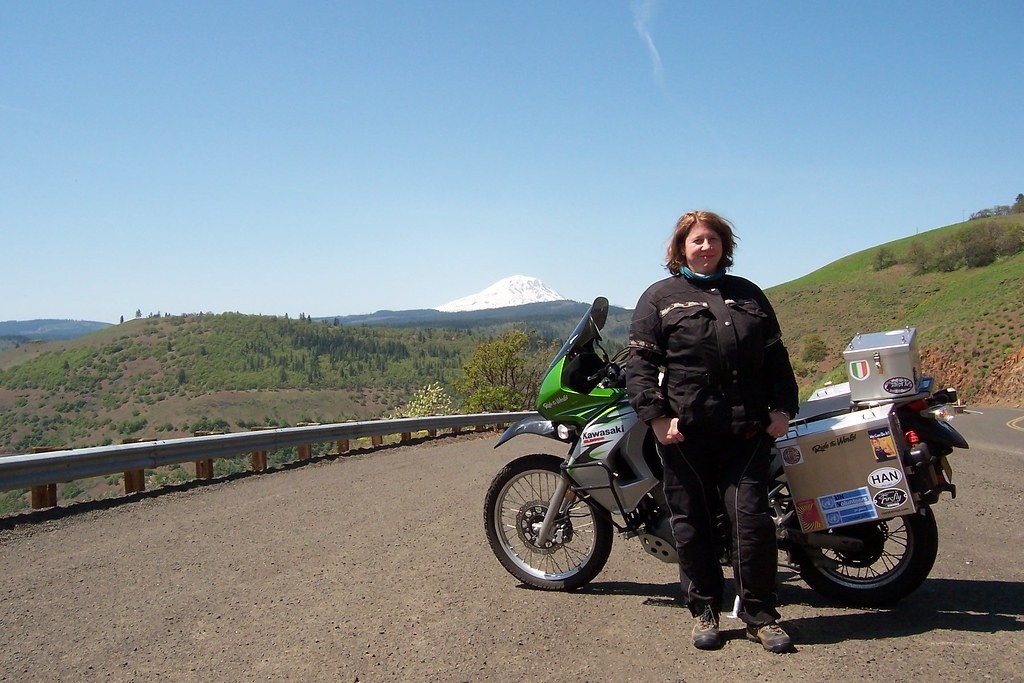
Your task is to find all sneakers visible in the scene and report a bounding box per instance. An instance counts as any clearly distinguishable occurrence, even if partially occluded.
[692,608,720,648]
[747,622,794,653]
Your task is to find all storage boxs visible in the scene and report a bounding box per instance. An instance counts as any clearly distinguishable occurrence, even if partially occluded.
[842,328,922,401]
[808,382,851,403]
[775,404,920,526]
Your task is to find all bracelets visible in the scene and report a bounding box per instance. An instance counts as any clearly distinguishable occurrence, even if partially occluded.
[775,408,791,419]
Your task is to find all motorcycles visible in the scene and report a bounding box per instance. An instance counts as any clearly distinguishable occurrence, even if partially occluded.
[483,296,968,608]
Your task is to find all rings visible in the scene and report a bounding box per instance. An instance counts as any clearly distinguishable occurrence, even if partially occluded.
[777,434,780,437]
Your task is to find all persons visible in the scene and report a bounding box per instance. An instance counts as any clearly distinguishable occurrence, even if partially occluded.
[625,211,800,651]
